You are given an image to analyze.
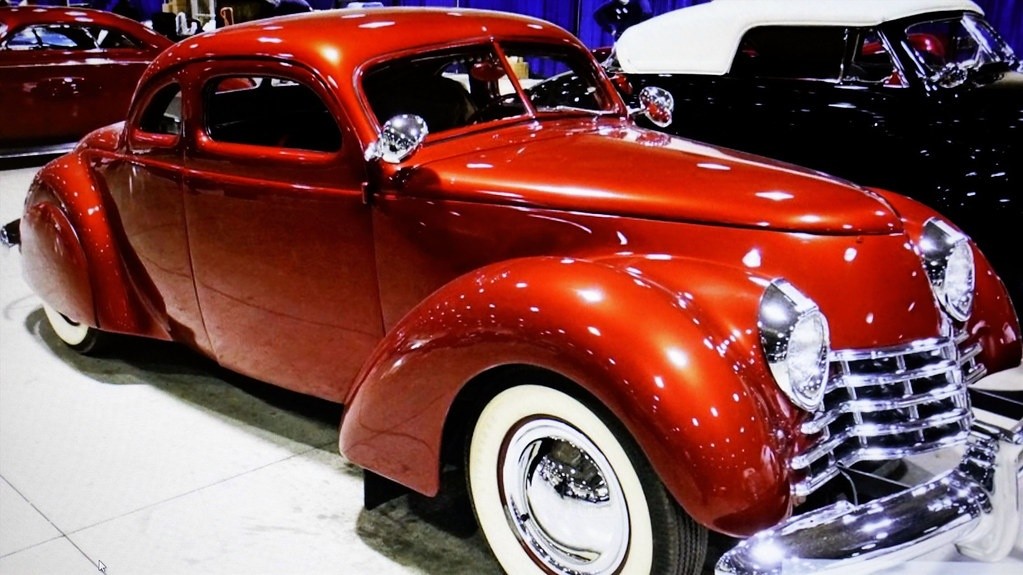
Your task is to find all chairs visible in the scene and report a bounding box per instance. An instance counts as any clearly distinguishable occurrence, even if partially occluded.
[383,60,481,130]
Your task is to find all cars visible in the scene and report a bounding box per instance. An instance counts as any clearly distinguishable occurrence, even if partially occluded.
[513,0,1023,315]
[0,6,260,161]
[0,6,1023,575]
